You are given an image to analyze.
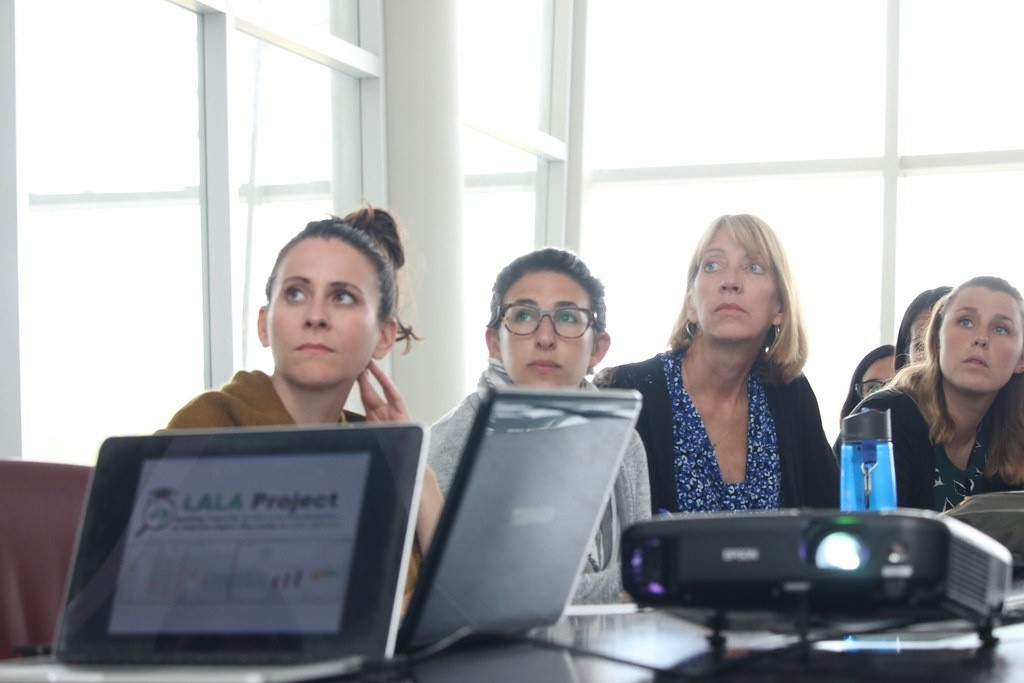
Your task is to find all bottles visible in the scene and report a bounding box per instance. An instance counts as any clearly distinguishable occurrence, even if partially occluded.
[839,408,897,509]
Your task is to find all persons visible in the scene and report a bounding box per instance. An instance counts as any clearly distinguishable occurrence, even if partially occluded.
[164,207,447,623]
[833,275,1024,513]
[419,247,654,606]
[594,215,841,516]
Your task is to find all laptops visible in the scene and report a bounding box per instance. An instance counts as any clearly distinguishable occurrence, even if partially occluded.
[0,417,430,683]
[387,388,643,672]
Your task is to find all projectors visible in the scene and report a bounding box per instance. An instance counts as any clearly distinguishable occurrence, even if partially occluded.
[618,509,1010,655]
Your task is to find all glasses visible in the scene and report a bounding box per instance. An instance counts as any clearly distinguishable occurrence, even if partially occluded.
[855,379,886,400]
[499,303,597,339]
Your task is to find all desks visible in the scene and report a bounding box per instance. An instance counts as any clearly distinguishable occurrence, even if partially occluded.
[309,570,1023,683]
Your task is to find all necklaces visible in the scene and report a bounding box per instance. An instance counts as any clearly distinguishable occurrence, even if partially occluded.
[685,349,741,447]
